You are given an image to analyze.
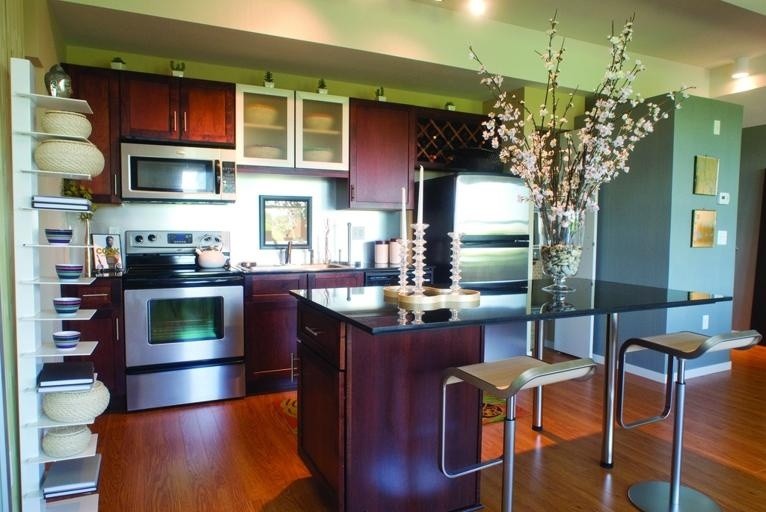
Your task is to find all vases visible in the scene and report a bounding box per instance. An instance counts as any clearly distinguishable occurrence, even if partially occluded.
[536,211,588,296]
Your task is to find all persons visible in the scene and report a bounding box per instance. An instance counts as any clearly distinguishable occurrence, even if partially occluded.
[105,236,121,276]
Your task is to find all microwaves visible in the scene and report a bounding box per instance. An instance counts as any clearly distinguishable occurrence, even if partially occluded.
[120,142,236,203]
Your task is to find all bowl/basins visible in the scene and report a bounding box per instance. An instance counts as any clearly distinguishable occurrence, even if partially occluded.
[54,264,83,281]
[52,330,81,351]
[43,229,73,244]
[247,145,282,158]
[303,145,339,161]
[246,102,279,125]
[52,297,80,315]
[305,115,335,131]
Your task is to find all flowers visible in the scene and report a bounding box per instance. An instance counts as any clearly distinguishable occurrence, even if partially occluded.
[464,6,699,245]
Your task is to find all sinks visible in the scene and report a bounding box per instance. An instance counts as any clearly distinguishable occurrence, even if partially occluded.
[232,263,354,272]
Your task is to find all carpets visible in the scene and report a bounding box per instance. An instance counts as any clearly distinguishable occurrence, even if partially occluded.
[270,392,532,441]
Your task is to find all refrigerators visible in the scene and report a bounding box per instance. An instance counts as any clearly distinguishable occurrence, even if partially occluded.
[412,172,530,362]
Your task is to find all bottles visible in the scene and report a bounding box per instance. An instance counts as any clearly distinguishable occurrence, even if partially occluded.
[389,238,401,264]
[374,240,389,264]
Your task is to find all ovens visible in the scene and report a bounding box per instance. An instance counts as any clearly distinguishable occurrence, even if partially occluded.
[123,284,246,371]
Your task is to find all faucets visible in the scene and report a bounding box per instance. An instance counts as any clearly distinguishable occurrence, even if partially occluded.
[284,241,294,265]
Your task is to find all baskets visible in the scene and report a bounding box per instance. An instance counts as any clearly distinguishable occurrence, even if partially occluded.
[34,139,106,177]
[42,425,91,458]
[42,380,111,422]
[42,110,92,139]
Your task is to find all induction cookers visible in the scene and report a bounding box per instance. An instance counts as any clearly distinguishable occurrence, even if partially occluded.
[123,264,237,279]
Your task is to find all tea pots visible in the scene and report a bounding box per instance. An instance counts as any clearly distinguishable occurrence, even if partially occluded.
[193,247,230,267]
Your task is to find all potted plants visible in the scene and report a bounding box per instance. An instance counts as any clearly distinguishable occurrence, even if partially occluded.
[263,68,276,87]
[444,101,456,112]
[375,82,387,102]
[166,58,186,78]
[109,56,126,70]
[317,77,328,94]
[61,178,97,277]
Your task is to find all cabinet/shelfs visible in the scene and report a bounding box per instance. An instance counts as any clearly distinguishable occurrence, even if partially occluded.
[413,105,504,174]
[62,278,124,404]
[348,97,415,210]
[117,71,236,145]
[245,272,364,382]
[236,83,349,176]
[8,55,104,512]
[61,62,117,204]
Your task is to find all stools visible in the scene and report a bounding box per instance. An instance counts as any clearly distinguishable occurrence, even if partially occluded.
[435,352,598,511]
[616,326,764,511]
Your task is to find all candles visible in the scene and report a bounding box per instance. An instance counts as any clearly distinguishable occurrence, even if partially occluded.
[417,163,425,223]
[400,182,407,241]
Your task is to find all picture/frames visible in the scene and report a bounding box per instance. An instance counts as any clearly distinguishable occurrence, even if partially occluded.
[90,233,124,277]
[692,154,721,197]
[258,194,314,251]
[690,209,717,248]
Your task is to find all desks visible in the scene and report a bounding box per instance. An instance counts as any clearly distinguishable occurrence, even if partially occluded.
[289,276,732,510]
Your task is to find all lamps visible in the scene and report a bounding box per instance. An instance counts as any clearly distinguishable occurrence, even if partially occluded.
[730,55,750,79]
